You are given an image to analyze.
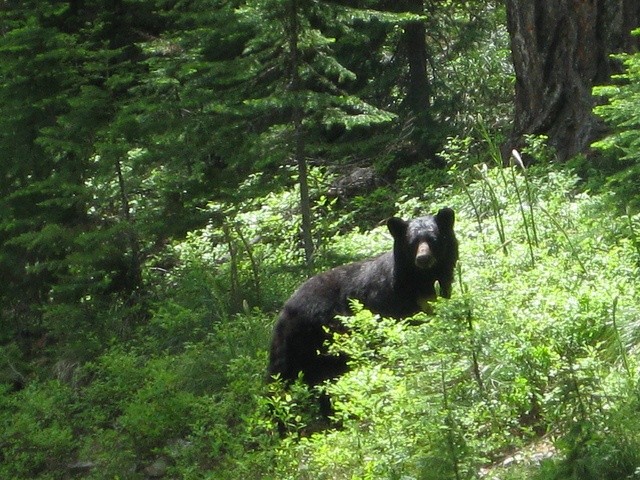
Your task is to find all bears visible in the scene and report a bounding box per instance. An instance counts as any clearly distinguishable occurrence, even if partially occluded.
[266,208,458,437]
